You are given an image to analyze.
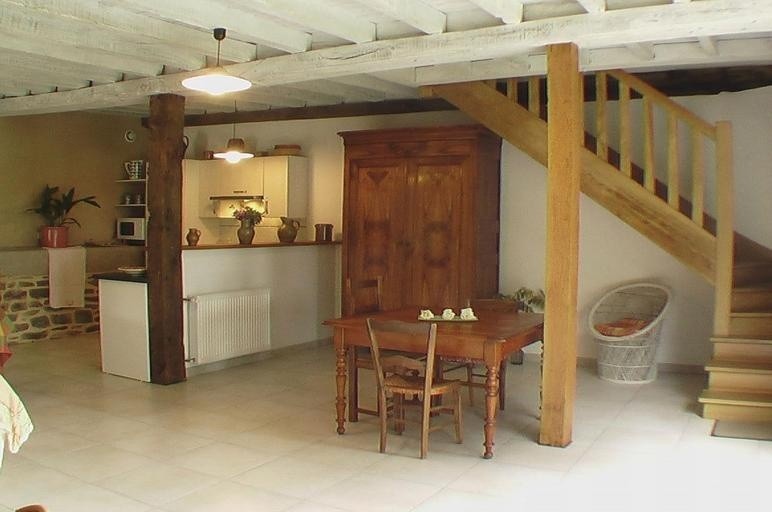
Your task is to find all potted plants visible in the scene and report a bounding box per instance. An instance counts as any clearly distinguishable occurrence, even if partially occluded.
[23,183,101,248]
[483,286,544,365]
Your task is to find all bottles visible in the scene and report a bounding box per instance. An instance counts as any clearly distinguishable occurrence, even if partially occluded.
[124,192,145,206]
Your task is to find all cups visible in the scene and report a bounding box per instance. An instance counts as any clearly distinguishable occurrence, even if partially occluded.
[124,159,144,180]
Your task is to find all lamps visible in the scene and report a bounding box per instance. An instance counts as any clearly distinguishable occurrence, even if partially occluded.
[212,99,255,165]
[180,28,253,97]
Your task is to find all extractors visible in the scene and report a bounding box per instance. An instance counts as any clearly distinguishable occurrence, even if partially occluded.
[208,195,265,201]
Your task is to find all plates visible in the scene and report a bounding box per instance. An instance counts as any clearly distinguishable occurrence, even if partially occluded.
[420,313,478,323]
[115,264,150,278]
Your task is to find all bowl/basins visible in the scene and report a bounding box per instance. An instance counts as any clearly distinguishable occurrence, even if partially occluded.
[315,224,333,240]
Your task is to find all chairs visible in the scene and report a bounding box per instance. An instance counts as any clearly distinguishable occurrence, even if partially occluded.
[434,299,520,417]
[364,318,462,459]
[587,281,671,384]
[344,277,439,422]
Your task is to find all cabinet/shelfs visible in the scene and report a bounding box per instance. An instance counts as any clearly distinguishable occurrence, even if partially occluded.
[195,160,221,218]
[264,156,307,227]
[337,123,501,319]
[221,157,265,195]
[115,162,148,219]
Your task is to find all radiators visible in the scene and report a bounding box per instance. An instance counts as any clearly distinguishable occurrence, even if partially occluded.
[182,288,271,366]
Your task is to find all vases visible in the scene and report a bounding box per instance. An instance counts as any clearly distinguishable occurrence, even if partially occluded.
[237,219,254,246]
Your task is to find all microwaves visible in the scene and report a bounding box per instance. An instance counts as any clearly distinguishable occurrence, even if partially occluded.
[115,217,146,241]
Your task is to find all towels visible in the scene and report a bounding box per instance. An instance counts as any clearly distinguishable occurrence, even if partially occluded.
[41,245,86,309]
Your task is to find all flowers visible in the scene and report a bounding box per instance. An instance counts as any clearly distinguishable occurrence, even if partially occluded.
[229,199,262,224]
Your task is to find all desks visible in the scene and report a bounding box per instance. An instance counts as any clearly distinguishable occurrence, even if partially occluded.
[321,305,544,460]
[89,271,150,384]
